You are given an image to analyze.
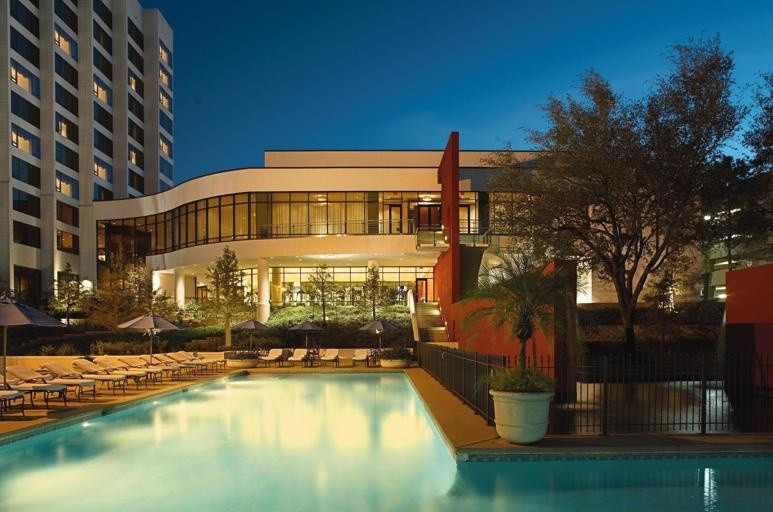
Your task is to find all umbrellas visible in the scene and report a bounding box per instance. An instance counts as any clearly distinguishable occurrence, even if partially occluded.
[288,320,323,348]
[356,317,403,351]
[116,309,183,378]
[0,290,68,410]
[233,318,269,351]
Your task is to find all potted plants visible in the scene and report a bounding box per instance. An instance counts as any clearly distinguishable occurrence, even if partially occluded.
[376,347,413,368]
[462,240,590,446]
[225,351,260,368]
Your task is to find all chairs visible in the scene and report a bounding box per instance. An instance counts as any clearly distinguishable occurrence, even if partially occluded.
[261,348,369,367]
[0,350,226,420]
